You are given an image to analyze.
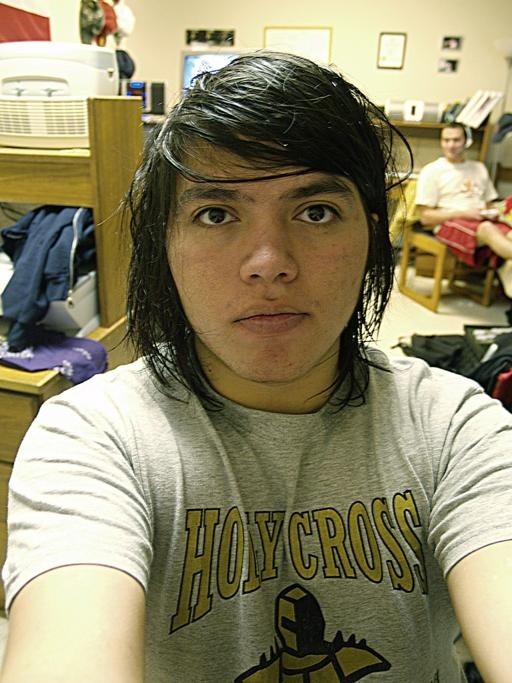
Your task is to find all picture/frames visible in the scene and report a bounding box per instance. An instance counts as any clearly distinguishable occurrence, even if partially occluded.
[178,25,407,106]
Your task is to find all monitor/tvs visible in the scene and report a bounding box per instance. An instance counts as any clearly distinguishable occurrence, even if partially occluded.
[180,49,241,96]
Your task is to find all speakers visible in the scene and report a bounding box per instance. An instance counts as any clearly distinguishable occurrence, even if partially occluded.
[151,83,164,114]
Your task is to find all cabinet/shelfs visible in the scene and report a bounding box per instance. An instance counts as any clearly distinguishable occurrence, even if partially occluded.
[362,107,492,224]
[0,93,166,575]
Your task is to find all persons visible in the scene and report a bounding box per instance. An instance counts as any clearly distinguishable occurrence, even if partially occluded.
[413,122,512,272]
[2,45,512,683]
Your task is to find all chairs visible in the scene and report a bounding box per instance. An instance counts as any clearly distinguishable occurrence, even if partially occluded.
[395,179,500,312]
